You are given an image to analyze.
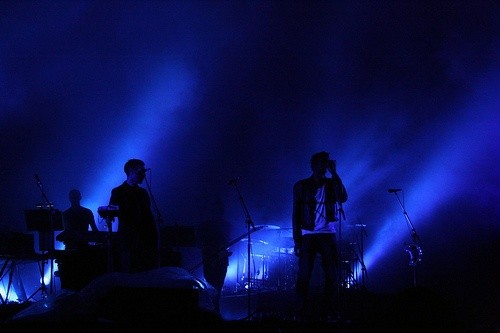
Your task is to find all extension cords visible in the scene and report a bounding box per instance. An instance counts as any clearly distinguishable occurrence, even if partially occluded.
[9,288,99,333]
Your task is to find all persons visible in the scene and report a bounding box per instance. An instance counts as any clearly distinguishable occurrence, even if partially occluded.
[292,152,348,298]
[198,204,232,314]
[109,159,172,272]
[63,189,99,238]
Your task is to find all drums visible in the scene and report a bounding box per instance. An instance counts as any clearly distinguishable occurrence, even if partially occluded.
[241,252,299,286]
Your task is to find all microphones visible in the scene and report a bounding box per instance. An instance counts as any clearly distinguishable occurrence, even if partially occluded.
[36,175,42,187]
[146,168,151,171]
[229,177,239,184]
[388,188,402,193]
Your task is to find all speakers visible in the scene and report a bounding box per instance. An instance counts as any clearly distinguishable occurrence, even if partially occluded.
[80,268,200,323]
[6,235,42,258]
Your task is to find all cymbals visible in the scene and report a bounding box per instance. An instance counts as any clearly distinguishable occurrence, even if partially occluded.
[240,238,268,245]
[247,224,281,231]
[269,236,295,248]
